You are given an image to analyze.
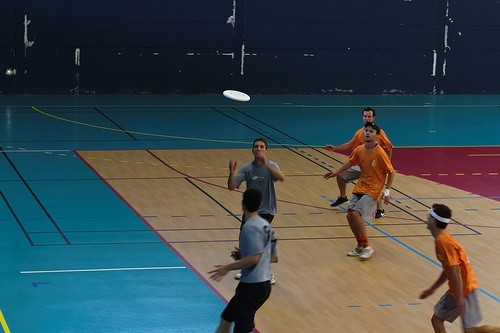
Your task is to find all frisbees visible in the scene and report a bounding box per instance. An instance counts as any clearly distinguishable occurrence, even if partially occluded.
[223,90,251,102]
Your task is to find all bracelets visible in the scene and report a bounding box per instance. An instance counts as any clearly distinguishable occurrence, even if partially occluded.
[383,188,390,196]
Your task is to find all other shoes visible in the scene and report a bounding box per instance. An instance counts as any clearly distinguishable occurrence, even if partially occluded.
[347,246,375,259]
[270,274,275,284]
[330,196,350,207]
[375,208,385,219]
[234,273,242,280]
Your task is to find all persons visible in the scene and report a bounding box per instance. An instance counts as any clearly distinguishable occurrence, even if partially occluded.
[326,108,394,219]
[207,188,280,333]
[418,203,500,333]
[227,138,285,284]
[324,122,395,258]
